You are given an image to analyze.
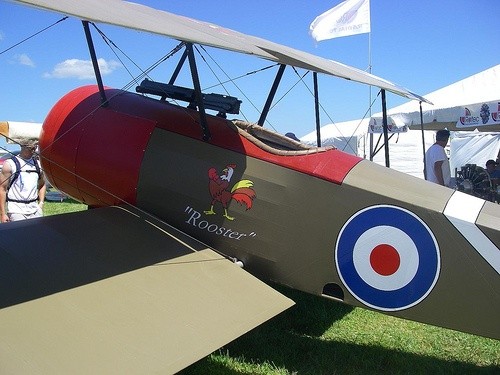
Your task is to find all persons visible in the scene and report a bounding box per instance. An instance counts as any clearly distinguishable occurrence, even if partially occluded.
[0,137,46,223]
[425,129,451,186]
[479,150,500,194]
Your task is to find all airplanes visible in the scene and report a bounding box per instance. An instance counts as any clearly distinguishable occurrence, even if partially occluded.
[1,0,499,375]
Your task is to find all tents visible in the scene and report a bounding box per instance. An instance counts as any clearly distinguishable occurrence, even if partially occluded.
[299,118,447,180]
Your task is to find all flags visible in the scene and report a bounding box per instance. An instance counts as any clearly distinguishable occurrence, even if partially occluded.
[310,0,370,40]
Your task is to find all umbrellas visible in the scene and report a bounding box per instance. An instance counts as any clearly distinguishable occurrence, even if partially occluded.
[367,66,500,132]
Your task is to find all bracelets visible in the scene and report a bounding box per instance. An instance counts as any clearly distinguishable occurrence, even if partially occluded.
[39,201,44,205]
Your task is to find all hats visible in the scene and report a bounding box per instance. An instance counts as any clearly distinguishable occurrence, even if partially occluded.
[486,160,498,166]
